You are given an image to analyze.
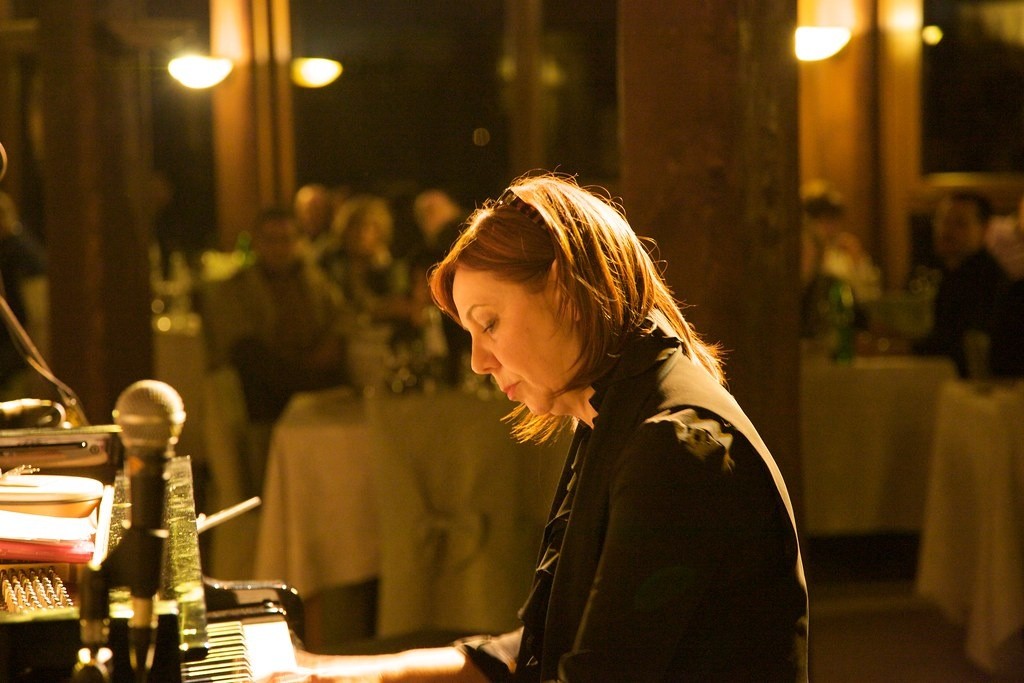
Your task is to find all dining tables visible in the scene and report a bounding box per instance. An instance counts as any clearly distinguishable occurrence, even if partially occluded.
[919,379,1024,683]
[271,382,581,633]
[798,341,954,581]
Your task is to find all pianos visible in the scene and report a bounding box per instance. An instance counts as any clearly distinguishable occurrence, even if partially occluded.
[5,437,311,683]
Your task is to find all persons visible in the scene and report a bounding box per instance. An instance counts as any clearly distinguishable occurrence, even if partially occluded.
[196,176,488,414]
[872,189,1022,391]
[797,179,884,307]
[247,171,811,682]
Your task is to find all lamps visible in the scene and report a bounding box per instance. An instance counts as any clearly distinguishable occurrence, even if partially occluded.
[795,0,855,63]
[167,54,234,90]
[289,57,345,88]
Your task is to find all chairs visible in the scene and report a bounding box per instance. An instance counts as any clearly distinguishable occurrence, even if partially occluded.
[200,358,275,594]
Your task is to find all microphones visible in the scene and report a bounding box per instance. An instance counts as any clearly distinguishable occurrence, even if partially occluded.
[111,379,186,670]
